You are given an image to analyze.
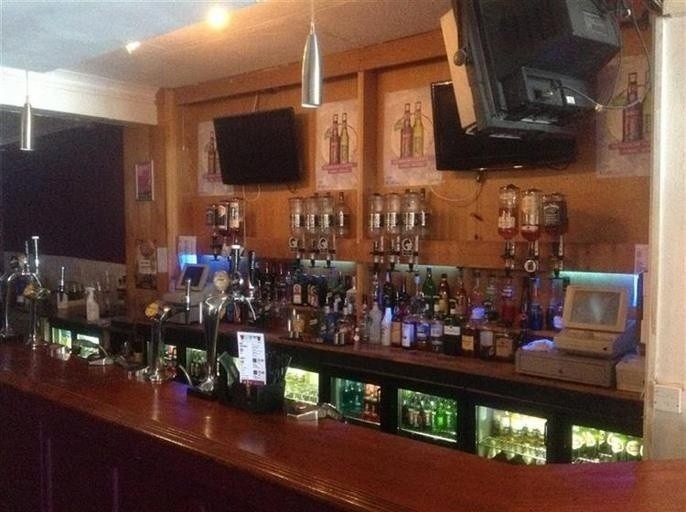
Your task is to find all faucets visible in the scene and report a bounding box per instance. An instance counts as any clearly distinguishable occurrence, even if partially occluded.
[205,293,258,379]
[0,271,44,338]
[146,282,193,363]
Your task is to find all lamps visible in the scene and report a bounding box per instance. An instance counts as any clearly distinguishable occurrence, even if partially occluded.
[301,1,322,109]
[19,69,34,152]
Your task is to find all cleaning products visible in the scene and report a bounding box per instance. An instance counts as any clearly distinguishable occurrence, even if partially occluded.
[85,286,99,320]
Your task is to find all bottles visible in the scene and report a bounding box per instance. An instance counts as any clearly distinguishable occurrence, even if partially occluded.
[361,188,435,255]
[331,378,458,445]
[277,189,351,250]
[204,193,245,262]
[399,102,424,160]
[329,112,350,165]
[622,66,652,144]
[205,130,216,176]
[492,184,567,260]
[201,250,568,365]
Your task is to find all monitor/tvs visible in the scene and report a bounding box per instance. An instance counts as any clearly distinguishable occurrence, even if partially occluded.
[441,0,624,135]
[212,107,303,185]
[177,263,208,291]
[428,79,575,171]
[562,284,629,332]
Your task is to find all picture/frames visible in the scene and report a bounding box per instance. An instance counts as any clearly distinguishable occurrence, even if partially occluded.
[135,160,154,201]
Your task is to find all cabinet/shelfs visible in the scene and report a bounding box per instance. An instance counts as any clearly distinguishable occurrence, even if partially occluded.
[47,299,644,465]
